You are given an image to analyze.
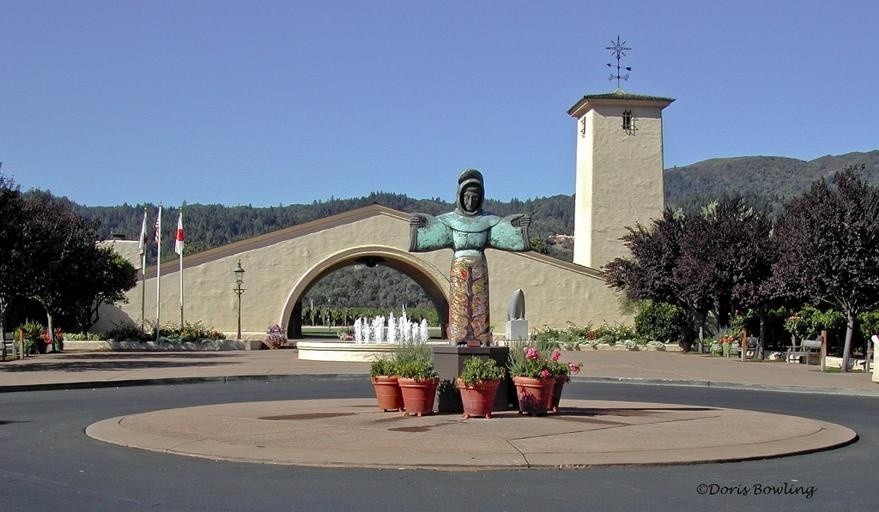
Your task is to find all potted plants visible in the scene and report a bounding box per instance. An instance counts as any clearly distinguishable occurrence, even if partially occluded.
[369,335,507,419]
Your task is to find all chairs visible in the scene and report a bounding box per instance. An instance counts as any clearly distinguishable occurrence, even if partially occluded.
[785,338,822,367]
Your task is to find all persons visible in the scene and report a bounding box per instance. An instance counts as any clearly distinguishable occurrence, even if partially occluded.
[408,169,531,347]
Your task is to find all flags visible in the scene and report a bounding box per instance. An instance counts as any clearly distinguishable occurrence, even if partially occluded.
[175,212,184,255]
[139,210,148,256]
[155,207,160,242]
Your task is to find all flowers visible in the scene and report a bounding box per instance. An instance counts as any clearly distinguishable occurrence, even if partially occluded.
[265,324,287,346]
[505,331,584,384]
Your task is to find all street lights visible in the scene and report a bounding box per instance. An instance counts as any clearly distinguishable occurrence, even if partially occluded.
[233,258,247,339]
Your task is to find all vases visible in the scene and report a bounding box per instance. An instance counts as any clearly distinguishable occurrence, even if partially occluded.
[513,374,565,416]
